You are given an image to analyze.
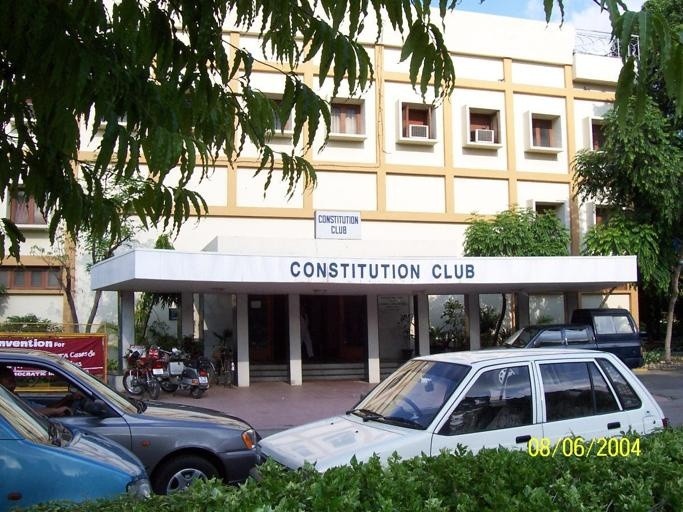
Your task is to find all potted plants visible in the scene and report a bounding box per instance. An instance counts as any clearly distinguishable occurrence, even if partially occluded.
[400,329,415,360]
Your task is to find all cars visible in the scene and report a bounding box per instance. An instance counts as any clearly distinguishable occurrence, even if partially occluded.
[249,346,670,492]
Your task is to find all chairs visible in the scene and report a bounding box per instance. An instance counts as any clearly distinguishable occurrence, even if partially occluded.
[445,374,530,435]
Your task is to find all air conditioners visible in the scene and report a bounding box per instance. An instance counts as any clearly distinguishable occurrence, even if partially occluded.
[405,124,429,138]
[471,129,495,143]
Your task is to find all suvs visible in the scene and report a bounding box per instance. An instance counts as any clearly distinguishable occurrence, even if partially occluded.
[478,306,645,371]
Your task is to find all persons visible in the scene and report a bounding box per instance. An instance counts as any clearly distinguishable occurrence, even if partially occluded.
[0,367,74,419]
[477,373,531,431]
[300,306,317,364]
